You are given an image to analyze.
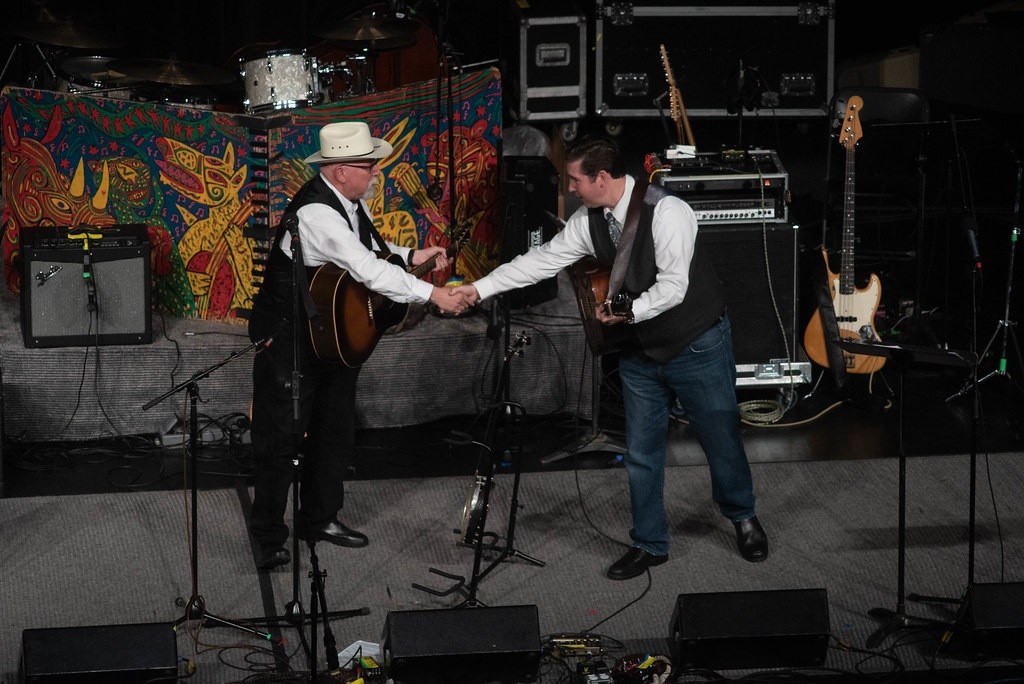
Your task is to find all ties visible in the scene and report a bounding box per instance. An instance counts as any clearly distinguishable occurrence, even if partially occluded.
[606,211,621,249]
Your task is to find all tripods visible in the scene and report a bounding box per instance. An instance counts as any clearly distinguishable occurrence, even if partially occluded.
[141,242,372,673]
[864,167,1024,650]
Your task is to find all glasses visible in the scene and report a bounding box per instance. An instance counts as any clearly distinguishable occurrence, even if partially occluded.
[340,159,380,175]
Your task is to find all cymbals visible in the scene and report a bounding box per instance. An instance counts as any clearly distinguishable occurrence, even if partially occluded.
[106,58,238,86]
[312,15,423,51]
[13,23,130,49]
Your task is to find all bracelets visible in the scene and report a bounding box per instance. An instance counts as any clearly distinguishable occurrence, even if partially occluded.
[408,249,415,266]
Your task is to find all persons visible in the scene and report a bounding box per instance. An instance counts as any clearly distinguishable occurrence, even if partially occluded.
[247,121,474,565]
[441,141,769,581]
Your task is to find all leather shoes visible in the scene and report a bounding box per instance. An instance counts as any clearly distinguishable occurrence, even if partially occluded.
[731,515,769,562]
[317,519,369,547]
[261,538,290,566]
[607,546,669,579]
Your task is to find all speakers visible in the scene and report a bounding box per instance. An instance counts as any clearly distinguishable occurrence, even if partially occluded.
[961,582,1024,660]
[669,586,830,671]
[382,602,543,684]
[697,223,797,369]
[17,621,177,684]
[16,227,153,347]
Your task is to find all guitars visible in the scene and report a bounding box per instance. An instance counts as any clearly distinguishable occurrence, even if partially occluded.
[803,94,888,376]
[573,268,636,359]
[307,224,471,369]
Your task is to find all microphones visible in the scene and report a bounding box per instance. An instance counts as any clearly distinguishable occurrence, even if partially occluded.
[84,245,97,313]
[285,215,299,245]
[265,314,289,349]
[964,229,982,270]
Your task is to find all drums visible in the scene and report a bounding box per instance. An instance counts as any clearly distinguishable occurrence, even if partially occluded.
[238,47,318,114]
[138,86,221,110]
[57,54,134,101]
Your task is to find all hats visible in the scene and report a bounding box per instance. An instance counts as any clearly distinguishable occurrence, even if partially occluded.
[304,122,394,163]
